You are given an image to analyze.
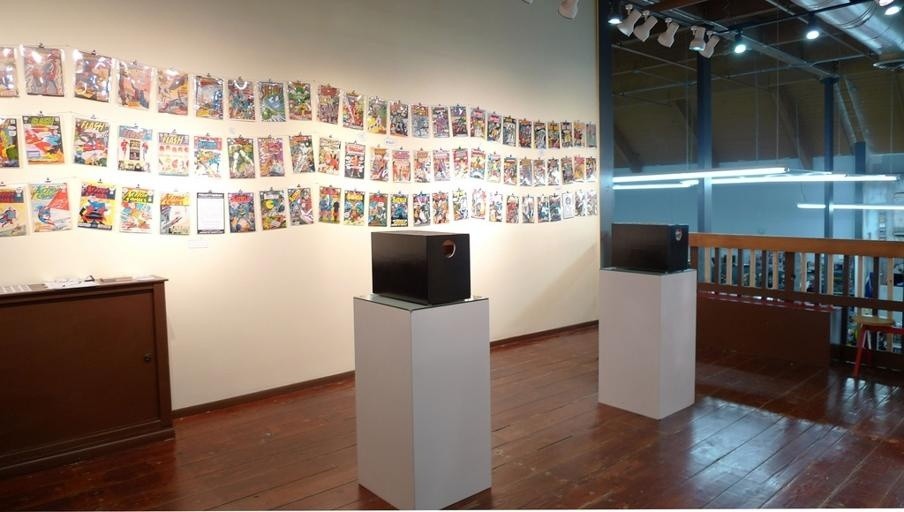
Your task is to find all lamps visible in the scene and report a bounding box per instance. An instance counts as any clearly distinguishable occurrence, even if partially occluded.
[555,0,721,59]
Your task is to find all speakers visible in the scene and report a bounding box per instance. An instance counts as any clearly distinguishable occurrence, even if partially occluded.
[611,223,689,272]
[371,230,472,303]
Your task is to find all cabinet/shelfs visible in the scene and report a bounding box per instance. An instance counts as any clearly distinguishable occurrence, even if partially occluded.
[0,274,176,479]
